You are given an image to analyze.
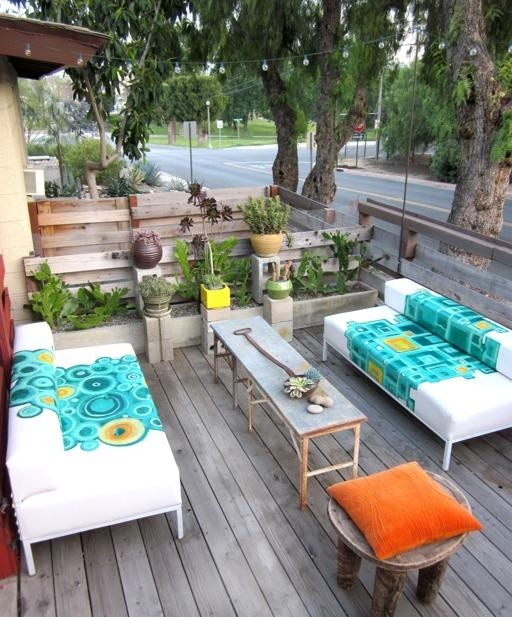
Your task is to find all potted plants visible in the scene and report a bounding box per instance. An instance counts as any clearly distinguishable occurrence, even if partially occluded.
[132,228,165,270]
[237,194,290,257]
[179,179,237,309]
[265,260,295,299]
[139,275,172,311]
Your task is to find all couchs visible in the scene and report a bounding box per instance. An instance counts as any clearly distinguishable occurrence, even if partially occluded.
[319,274,512,471]
[6,313,185,575]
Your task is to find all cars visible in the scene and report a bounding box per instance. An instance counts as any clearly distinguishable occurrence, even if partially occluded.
[35,133,90,146]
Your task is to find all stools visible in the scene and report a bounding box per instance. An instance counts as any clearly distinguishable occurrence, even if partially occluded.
[323,472,471,616]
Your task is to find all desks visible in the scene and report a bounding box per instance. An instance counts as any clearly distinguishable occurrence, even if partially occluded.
[208,314,367,509]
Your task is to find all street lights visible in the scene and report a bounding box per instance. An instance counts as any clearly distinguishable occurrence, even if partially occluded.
[204,100,213,148]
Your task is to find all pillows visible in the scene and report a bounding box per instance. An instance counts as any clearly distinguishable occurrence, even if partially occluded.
[325,458,484,560]
